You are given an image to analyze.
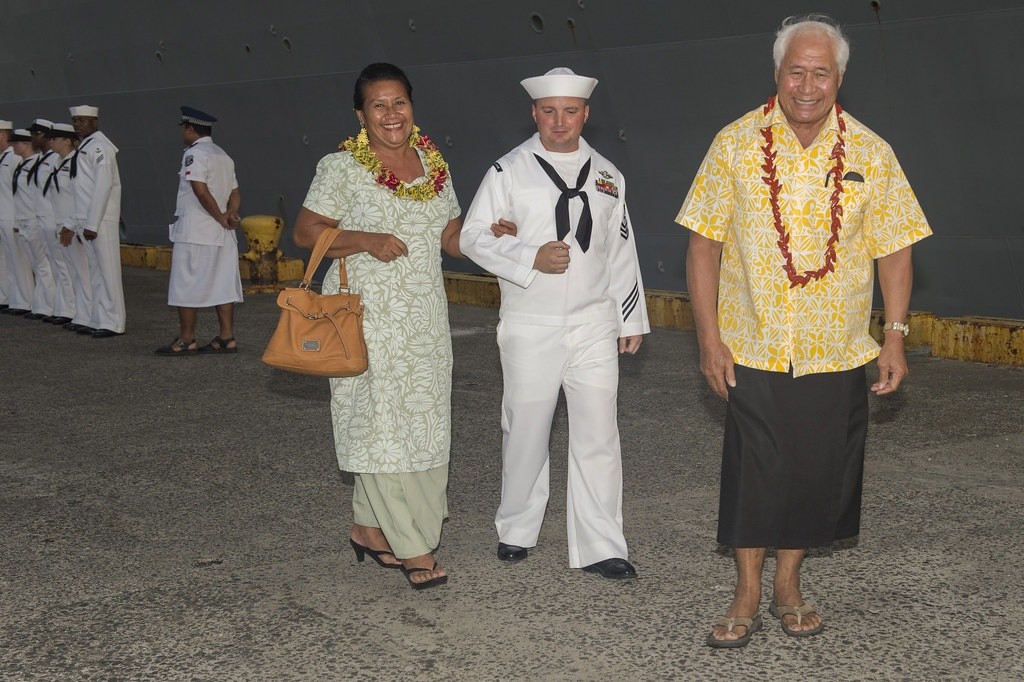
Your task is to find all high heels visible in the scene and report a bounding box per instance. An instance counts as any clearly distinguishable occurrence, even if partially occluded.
[401,554,447,590]
[350,538,404,568]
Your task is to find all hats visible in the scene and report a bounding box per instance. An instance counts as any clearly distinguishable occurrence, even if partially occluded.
[519,67,599,99]
[69,104,99,118]
[7,128,32,142]
[179,106,218,136]
[0,119,13,129]
[26,119,54,136]
[43,123,79,139]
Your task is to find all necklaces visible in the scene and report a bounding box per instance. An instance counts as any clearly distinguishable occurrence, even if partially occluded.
[759,96,847,290]
[337,123,451,202]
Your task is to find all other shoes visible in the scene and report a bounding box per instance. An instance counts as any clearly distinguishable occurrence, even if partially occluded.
[0,304,126,338]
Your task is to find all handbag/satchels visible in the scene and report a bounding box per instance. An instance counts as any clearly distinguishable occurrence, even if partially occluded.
[261,228,368,378]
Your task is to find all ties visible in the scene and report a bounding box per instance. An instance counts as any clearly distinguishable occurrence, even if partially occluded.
[12,159,33,197]
[43,159,70,198]
[0,152,9,165]
[533,152,593,254]
[27,152,54,187]
[70,138,92,179]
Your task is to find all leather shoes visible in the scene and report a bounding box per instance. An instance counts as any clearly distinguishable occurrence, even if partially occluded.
[498,541,526,561]
[581,558,638,579]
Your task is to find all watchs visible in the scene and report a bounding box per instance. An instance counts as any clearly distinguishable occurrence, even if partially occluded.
[882,322,910,337]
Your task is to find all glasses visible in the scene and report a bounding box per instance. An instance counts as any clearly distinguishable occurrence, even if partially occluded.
[31,131,43,136]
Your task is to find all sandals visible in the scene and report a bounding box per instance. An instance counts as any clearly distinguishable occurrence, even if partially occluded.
[198,335,238,355]
[153,336,199,356]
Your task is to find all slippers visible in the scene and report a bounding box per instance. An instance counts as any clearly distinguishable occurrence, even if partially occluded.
[768,597,823,637]
[706,612,763,649]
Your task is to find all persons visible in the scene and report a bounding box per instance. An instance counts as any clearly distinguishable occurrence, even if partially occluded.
[0,105,127,338]
[293,62,518,589]
[675,20,934,647]
[155,107,242,357]
[458,67,651,578]
